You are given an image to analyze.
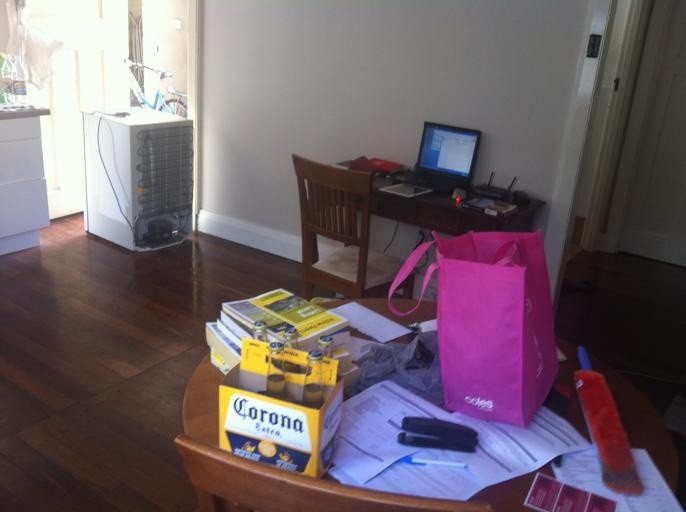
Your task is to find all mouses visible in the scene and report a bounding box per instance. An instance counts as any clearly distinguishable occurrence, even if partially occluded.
[451,186,467,202]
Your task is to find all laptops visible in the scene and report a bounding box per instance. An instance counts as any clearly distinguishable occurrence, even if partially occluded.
[385,121,483,197]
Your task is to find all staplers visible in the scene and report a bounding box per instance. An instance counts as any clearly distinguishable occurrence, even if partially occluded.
[397,417,479,453]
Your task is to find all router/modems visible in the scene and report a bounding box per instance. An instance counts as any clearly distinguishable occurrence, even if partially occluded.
[474,170,516,200]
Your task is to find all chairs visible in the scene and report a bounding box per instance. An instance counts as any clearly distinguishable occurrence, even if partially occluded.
[292,153,421,300]
[175,432,493,511]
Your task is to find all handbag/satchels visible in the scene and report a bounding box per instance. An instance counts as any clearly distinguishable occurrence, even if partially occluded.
[436,231,560,428]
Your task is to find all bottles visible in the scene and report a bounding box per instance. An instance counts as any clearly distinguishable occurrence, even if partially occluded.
[0,52,28,111]
[253,318,334,409]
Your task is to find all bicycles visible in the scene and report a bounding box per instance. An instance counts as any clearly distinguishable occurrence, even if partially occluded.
[125,59,187,117]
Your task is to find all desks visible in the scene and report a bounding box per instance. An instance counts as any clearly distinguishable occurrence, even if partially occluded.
[336,157,546,237]
[182,300,621,512]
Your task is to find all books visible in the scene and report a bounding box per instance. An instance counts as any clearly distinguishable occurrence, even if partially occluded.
[204,288,361,389]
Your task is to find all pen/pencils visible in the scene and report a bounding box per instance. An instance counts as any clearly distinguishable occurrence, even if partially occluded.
[398,456,468,468]
